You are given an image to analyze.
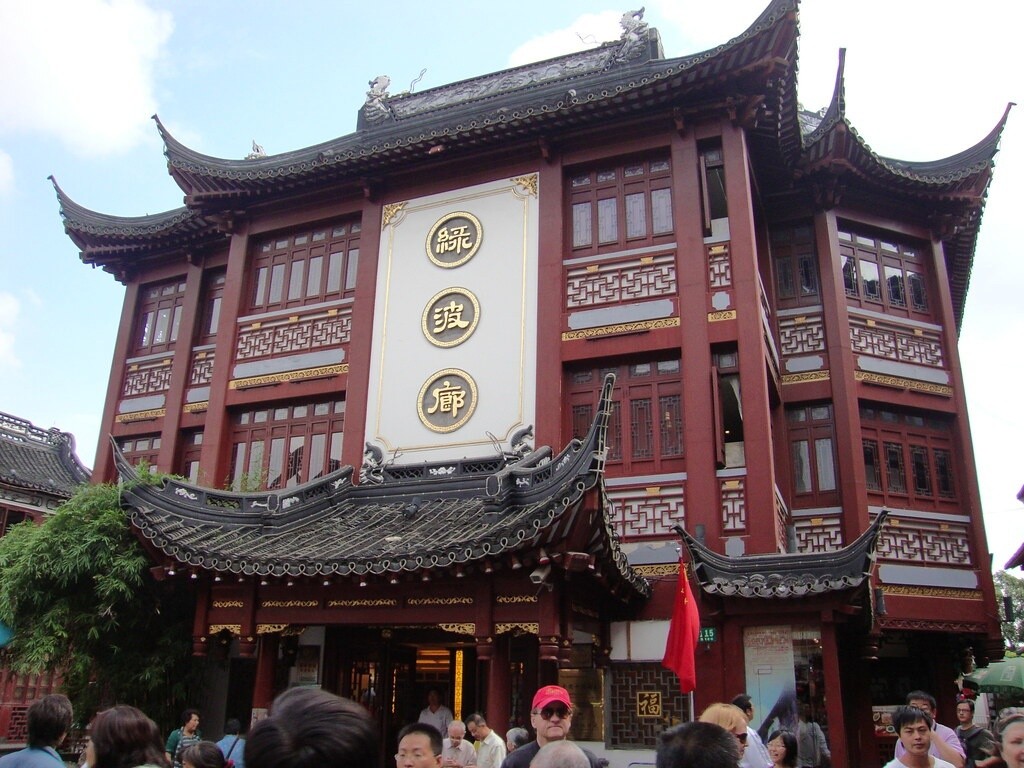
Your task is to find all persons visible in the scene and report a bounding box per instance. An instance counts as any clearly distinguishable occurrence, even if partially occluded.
[0,681,1024,768]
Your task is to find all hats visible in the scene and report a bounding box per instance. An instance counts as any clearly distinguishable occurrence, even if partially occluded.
[994,707,1024,734]
[532,685,572,712]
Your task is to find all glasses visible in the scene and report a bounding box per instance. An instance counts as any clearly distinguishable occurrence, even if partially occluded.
[394,754,438,762]
[956,708,971,713]
[909,704,932,713]
[767,743,785,748]
[735,732,748,744]
[533,707,570,720]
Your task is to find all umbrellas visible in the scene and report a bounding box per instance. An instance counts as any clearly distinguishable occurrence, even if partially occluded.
[962,656,1024,696]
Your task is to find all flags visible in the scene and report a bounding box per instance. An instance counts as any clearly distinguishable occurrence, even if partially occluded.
[661,558,700,695]
